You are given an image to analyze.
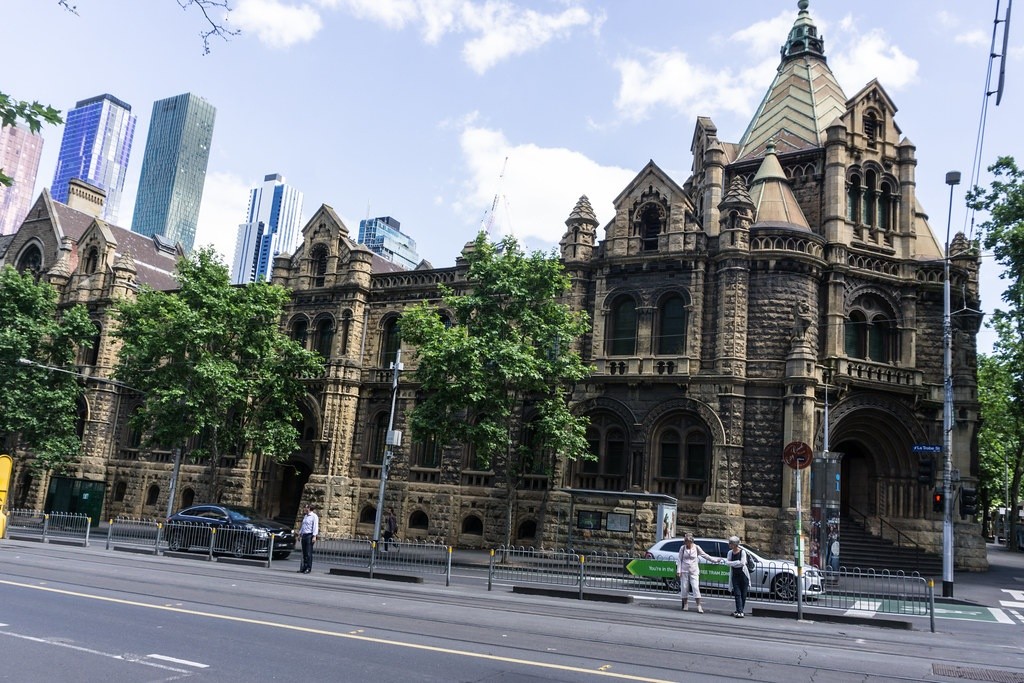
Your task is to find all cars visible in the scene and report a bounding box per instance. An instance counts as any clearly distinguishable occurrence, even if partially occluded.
[164,505,295,560]
[643,538,826,601]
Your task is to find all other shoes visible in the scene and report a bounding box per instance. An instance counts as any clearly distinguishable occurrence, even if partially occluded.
[731,612,744,618]
[297,570,304,573]
[683,604,688,610]
[304,568,310,574]
[697,606,703,613]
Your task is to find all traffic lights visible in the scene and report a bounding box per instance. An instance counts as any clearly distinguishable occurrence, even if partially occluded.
[959,486,979,521]
[933,489,945,511]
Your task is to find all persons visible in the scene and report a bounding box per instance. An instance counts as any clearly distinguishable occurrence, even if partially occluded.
[721,536,751,618]
[663,511,675,539]
[677,533,720,613]
[297,505,318,574]
[384,508,396,552]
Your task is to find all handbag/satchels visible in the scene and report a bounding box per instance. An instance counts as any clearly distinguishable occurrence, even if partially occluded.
[740,549,756,573]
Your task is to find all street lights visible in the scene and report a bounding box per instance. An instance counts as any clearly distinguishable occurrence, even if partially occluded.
[369,362,405,552]
[944,172,961,598]
